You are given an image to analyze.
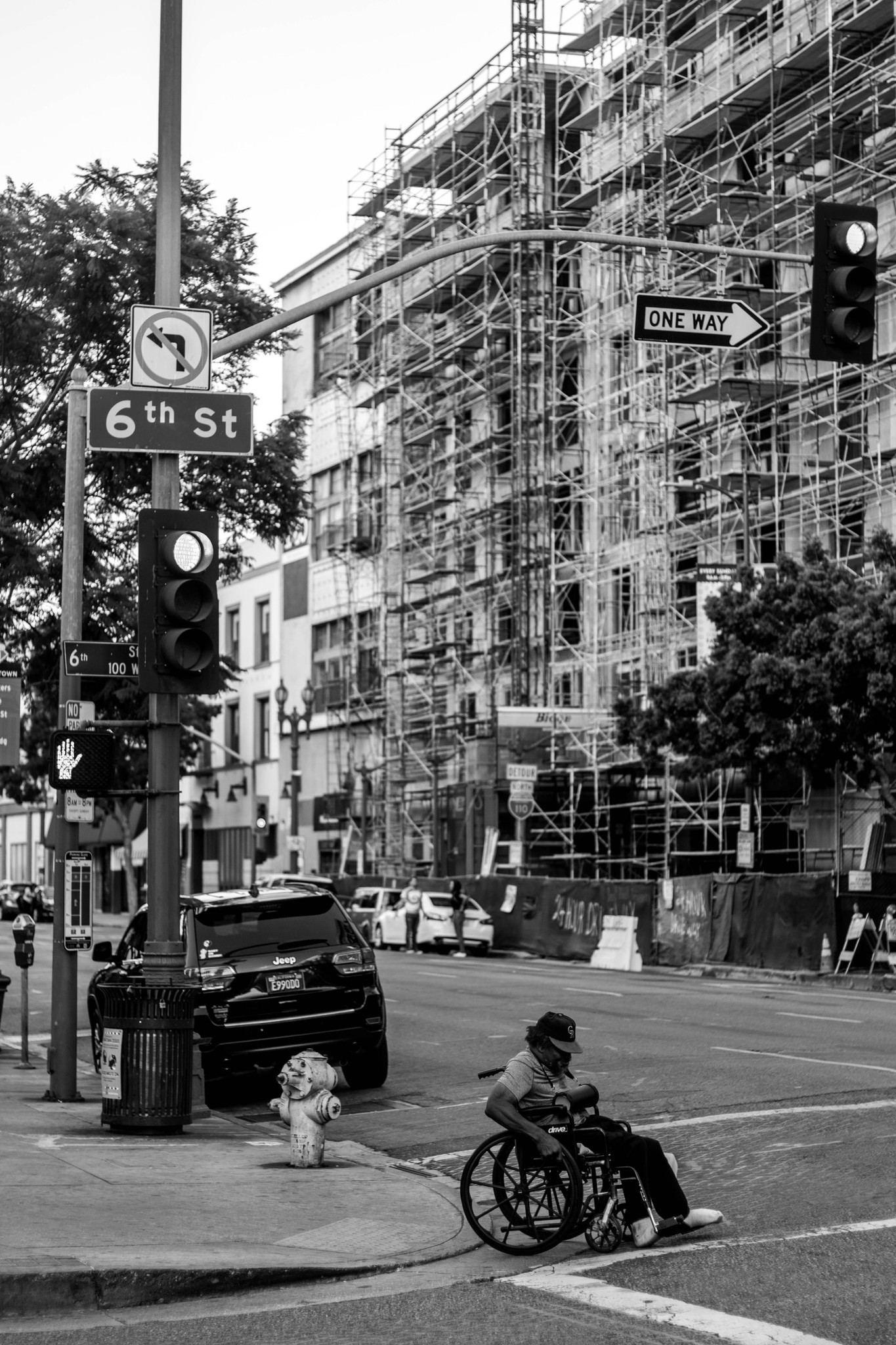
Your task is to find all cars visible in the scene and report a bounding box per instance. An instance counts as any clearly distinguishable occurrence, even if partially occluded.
[0,879,42,920]
[345,886,407,946]
[373,890,496,956]
[37,885,56,917]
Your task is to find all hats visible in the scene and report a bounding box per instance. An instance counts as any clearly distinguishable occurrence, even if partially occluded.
[536,1012,584,1053]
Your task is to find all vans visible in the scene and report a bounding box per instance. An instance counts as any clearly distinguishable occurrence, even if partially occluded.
[252,874,335,901]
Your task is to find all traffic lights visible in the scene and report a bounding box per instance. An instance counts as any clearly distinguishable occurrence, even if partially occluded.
[810,205,884,367]
[137,509,231,700]
[49,733,114,789]
[252,796,271,836]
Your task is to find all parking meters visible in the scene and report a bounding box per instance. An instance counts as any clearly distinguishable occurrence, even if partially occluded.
[12,915,36,1070]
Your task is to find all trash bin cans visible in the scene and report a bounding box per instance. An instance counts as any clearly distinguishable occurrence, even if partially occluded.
[97,983,203,1136]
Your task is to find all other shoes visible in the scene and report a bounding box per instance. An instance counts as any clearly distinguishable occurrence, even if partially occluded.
[416,950,423,954]
[454,952,467,958]
[406,950,414,953]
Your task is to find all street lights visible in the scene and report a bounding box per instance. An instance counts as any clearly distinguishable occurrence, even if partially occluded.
[275,677,316,873]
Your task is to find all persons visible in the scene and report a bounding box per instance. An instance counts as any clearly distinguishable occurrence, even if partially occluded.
[449,879,470,959]
[15,882,42,923]
[400,877,423,955]
[484,1011,724,1248]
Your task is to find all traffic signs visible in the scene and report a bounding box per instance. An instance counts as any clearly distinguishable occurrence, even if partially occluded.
[630,291,773,350]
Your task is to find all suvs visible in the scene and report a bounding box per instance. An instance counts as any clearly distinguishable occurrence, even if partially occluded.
[87,885,390,1102]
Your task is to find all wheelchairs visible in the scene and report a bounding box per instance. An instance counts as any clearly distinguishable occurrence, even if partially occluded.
[458,1065,666,1256]
